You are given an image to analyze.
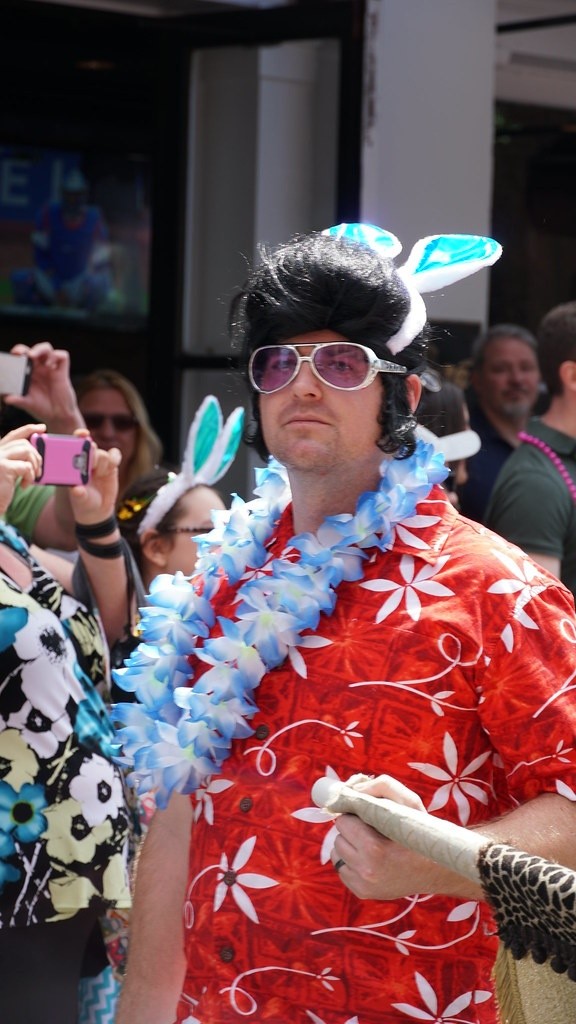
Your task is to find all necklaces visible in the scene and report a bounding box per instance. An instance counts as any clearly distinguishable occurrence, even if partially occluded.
[517,429,576,505]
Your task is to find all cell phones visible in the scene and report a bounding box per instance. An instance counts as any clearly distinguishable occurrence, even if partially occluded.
[30,432,93,486]
[0,352,32,398]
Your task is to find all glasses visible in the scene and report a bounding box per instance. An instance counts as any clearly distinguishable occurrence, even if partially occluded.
[79,414,139,432]
[247,343,406,396]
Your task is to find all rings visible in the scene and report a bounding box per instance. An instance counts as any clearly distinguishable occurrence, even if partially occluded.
[334,859,346,875]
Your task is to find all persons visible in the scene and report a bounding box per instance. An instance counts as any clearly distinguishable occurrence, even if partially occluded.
[0,342,104,552]
[0,420,135,1024]
[457,317,541,523]
[113,471,230,593]
[74,368,160,519]
[484,305,576,594]
[413,380,471,508]
[115,229,576,1024]
